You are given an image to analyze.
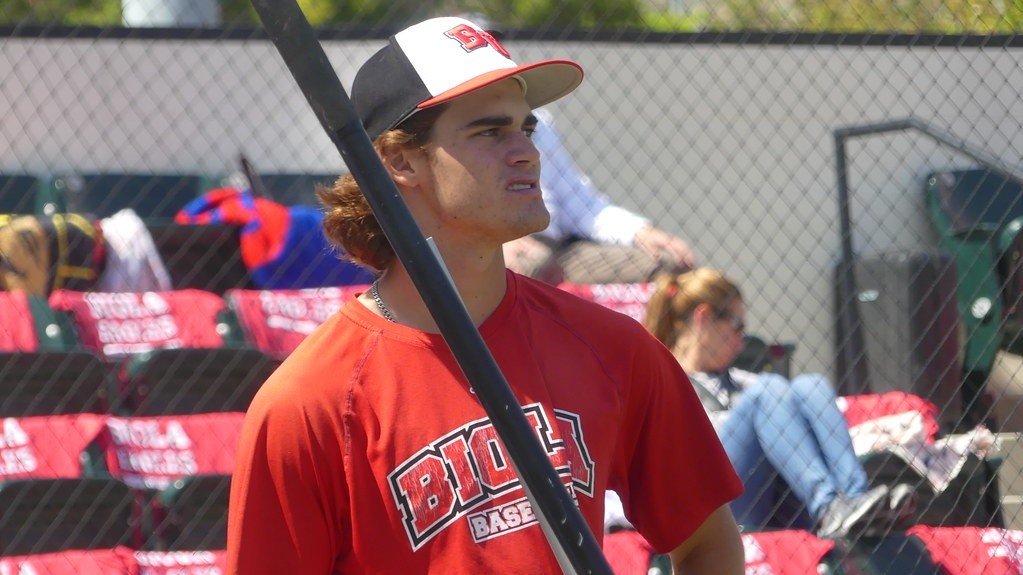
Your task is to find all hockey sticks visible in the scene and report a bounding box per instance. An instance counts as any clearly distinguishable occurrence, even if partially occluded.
[246,0,615,575]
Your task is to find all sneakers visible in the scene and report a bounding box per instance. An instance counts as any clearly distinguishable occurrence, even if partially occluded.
[819,482,888,538]
[888,485,917,527]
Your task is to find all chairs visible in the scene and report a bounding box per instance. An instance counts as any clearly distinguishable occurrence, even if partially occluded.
[0,170,1023,575]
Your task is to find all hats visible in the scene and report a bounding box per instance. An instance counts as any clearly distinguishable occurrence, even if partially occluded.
[352,15,585,146]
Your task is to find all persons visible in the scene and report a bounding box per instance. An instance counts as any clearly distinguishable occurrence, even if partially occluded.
[225,16,744,575]
[484,28,696,286]
[644,267,917,539]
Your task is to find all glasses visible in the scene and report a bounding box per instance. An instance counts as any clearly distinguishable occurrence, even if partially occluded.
[724,315,745,334]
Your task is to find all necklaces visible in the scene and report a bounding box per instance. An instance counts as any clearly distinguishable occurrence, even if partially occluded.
[372,282,397,324]
